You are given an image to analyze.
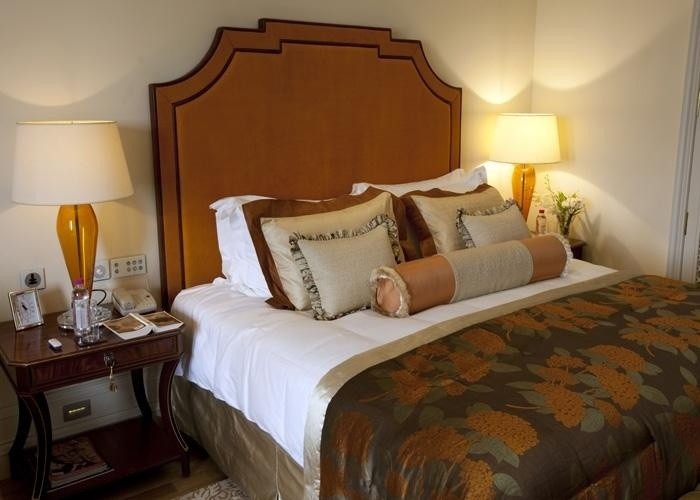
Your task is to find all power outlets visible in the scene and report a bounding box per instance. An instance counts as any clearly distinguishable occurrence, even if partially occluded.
[19,268,45,291]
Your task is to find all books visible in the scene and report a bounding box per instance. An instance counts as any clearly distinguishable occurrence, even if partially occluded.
[46,437,113,494]
[104,311,184,340]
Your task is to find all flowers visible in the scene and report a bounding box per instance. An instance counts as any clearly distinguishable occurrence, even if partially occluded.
[548,191,583,233]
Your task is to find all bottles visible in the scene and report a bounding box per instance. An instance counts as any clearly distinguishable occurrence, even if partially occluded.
[536,208,547,236]
[72,277,92,336]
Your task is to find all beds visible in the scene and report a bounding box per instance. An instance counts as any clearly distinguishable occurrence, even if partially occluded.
[149,19,700,500]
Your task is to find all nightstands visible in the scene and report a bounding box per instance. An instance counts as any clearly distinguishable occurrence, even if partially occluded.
[570,237,586,261]
[0,301,188,499]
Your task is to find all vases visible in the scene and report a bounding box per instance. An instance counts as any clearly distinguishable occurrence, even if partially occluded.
[559,221,570,238]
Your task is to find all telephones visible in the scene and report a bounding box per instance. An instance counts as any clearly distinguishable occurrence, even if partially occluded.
[111,286,157,316]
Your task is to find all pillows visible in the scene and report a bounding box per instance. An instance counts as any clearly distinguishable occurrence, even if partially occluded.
[208,195,334,297]
[351,169,488,195]
[289,214,403,322]
[455,200,532,249]
[399,182,504,258]
[242,186,416,311]
[370,234,569,319]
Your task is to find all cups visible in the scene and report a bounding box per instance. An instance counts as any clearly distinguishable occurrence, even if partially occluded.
[80,300,100,342]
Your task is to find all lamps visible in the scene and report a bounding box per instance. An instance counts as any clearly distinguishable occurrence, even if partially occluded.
[490,111,560,222]
[10,121,134,332]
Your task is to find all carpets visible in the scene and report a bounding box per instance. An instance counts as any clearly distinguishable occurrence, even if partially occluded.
[182,477,700,500]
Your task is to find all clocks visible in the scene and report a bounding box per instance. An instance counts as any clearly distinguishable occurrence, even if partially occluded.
[8,288,45,331]
